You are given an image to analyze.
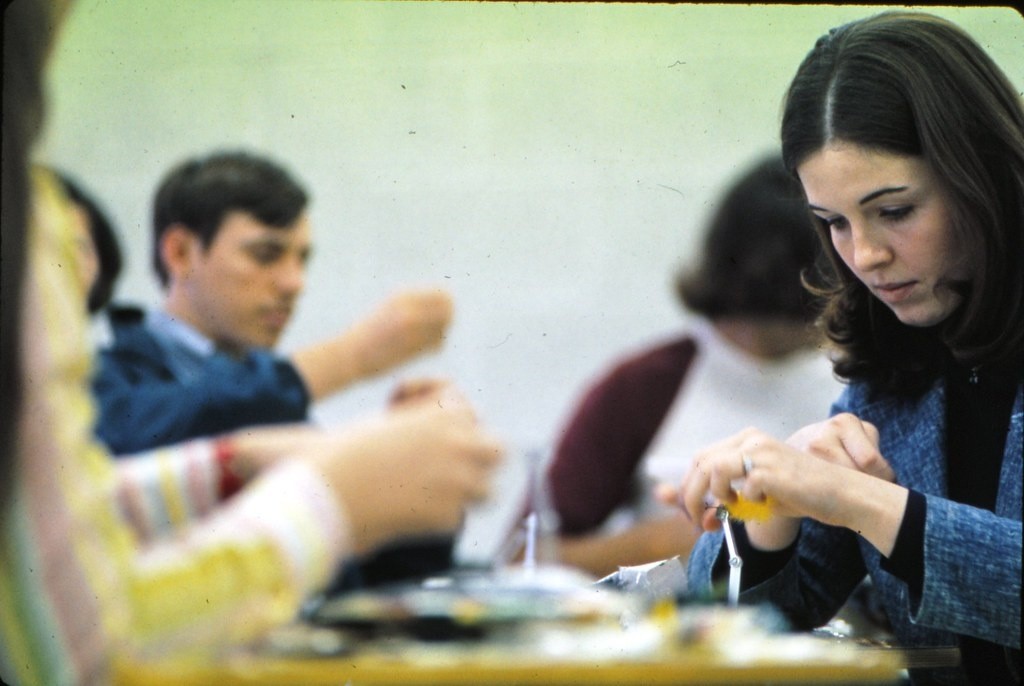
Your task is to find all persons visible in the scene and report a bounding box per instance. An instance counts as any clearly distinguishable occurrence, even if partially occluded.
[0,0,501,685]
[655,9,1024,686]
[88,151,457,601]
[513,156,849,581]
